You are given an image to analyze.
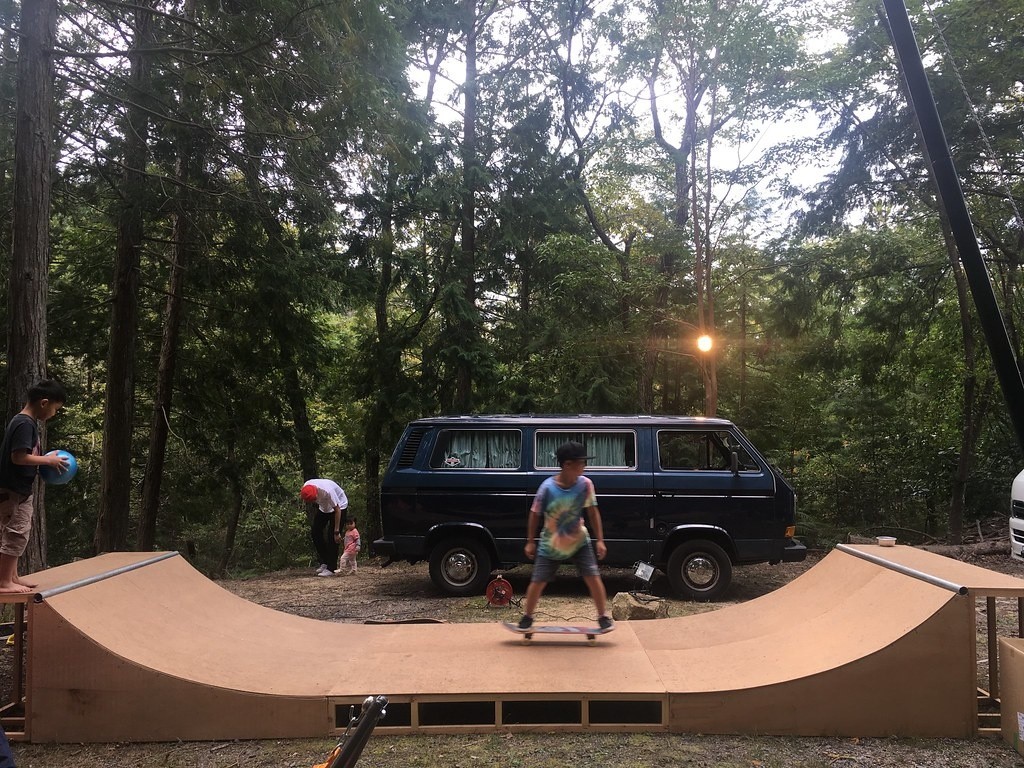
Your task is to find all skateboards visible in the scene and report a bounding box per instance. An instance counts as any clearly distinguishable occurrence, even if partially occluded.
[502,619,615,647]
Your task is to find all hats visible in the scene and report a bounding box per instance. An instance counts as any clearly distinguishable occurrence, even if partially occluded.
[557,442,597,462]
[301,485,318,501]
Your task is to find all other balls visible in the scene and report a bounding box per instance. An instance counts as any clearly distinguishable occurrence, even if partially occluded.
[41,450,77,485]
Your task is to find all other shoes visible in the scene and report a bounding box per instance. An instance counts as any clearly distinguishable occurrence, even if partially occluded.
[316,564,326,572]
[334,568,344,573]
[318,569,334,576]
[347,570,357,575]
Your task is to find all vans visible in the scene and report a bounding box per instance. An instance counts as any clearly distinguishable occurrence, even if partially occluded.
[365,415,807,603]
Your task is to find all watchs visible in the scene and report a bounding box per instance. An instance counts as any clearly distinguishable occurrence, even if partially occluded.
[334,531,340,535]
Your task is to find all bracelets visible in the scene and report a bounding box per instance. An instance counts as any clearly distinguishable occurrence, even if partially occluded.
[357,545,361,546]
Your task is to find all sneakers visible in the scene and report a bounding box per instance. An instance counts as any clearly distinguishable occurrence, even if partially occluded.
[599,617,613,633]
[518,616,533,632]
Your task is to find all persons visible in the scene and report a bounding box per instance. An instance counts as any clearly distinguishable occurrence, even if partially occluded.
[300,479,348,577]
[333,516,362,576]
[518,441,613,633]
[0,380,71,593]
